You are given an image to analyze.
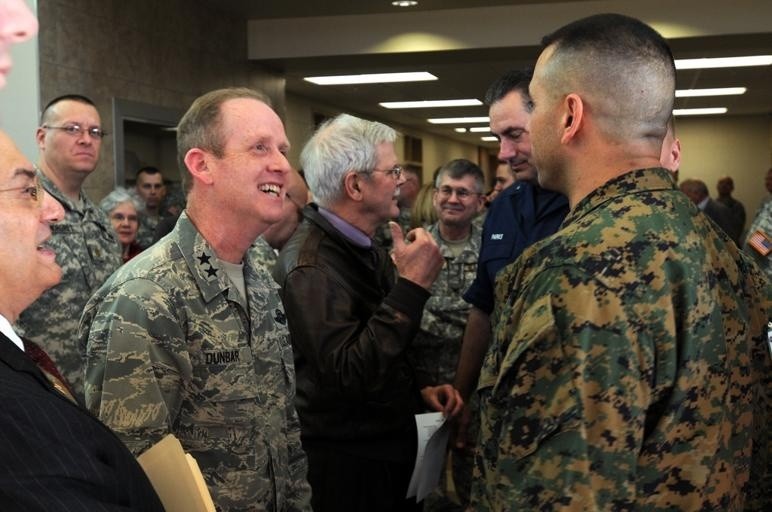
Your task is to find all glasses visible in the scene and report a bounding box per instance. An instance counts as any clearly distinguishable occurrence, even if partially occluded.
[0,178,46,203]
[40,124,107,141]
[372,165,403,180]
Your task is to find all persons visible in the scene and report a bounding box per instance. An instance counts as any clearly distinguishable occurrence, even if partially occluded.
[454,63,570,433]
[378,158,514,405]
[98,168,181,265]
[273,111,463,511]
[76,86,313,512]
[660,111,682,174]
[0,0,39,87]
[463,11,770,512]
[683,171,771,268]
[1,125,168,510]
[9,93,121,407]
[259,167,312,250]
